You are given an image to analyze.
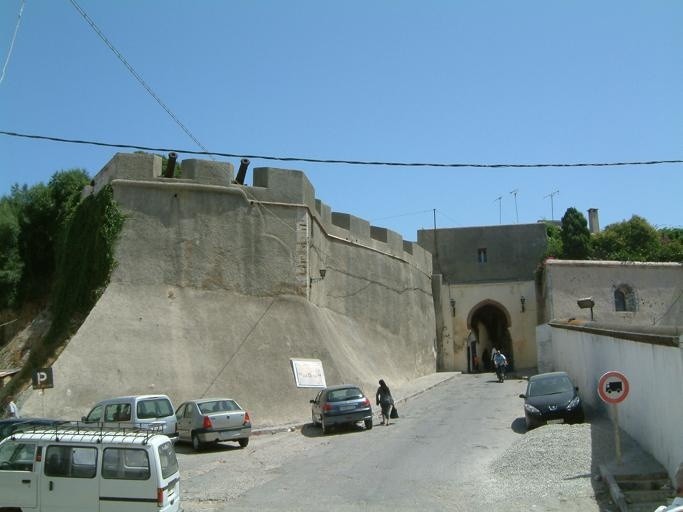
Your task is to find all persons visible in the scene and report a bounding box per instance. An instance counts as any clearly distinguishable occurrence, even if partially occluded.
[4,396,18,418]
[375,379,393,426]
[494,350,507,382]
[488,345,500,373]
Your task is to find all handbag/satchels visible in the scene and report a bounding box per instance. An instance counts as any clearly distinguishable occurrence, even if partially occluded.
[390,408,398,418]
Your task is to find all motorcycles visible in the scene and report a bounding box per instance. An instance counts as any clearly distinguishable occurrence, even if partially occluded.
[496,361,508,382]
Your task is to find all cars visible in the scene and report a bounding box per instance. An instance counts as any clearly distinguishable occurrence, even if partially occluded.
[0,394,251,512]
[519,372,583,428]
[310,385,372,434]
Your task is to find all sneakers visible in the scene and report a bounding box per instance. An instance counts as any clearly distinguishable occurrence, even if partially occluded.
[381,422,389,426]
